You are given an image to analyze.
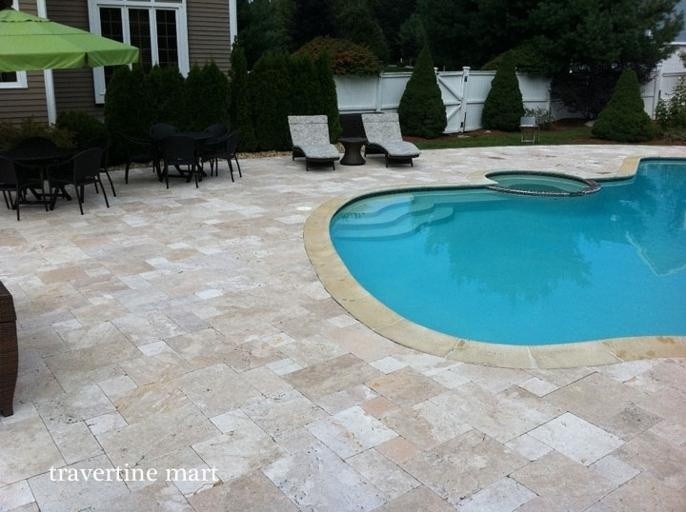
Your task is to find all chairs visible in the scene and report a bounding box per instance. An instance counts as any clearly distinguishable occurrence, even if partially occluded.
[519,117,541,144]
[0,122,241,221]
[362,114,420,167]
[287,114,340,171]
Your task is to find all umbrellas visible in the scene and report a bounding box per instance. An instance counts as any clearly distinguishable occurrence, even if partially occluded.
[0,8,142,70]
